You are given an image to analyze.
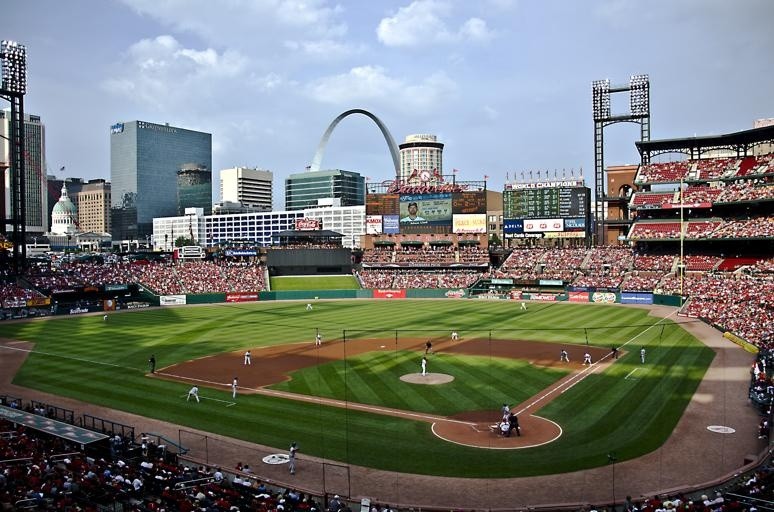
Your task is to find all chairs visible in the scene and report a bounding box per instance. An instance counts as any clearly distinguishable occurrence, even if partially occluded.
[628,155,774,274]
[0,419,313,512]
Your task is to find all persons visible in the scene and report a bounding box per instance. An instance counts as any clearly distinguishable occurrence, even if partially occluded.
[243,350,252,365]
[1,399,399,512]
[315,332,322,346]
[425,340,433,354]
[352,244,774,295]
[560,346,646,366]
[186,384,200,404]
[630,152,774,239]
[686,293,774,399]
[452,331,458,342]
[400,202,428,224]
[148,354,156,373]
[231,376,239,399]
[0,259,264,310]
[421,356,427,376]
[601,399,774,512]
